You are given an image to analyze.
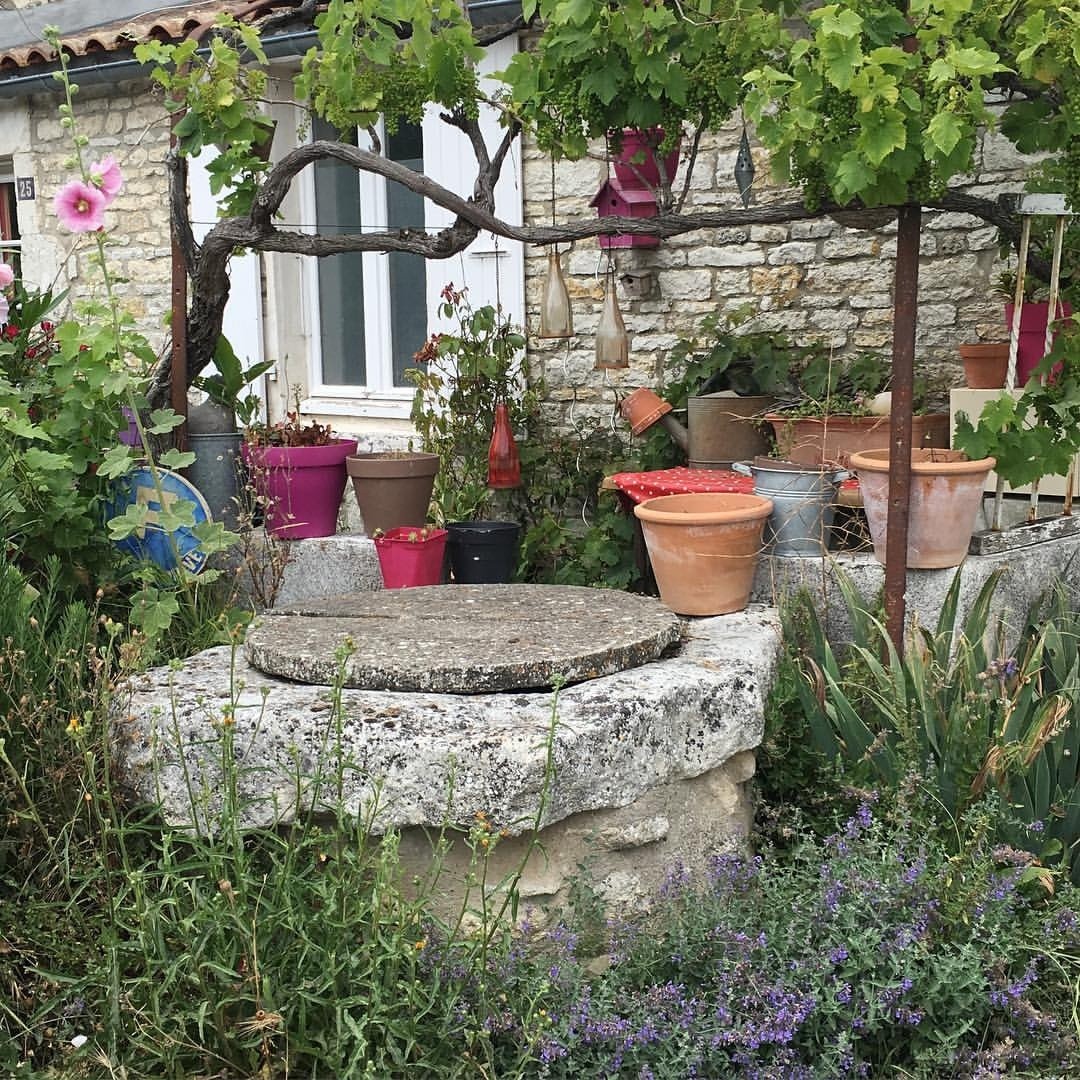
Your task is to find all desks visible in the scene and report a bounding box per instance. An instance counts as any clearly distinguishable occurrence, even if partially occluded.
[603,466,864,597]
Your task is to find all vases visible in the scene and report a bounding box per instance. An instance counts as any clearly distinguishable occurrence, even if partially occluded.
[604,126,682,191]
[187,425,525,591]
[622,338,1009,619]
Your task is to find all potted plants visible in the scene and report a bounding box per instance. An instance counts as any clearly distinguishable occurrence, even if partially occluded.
[990,266,1075,388]
